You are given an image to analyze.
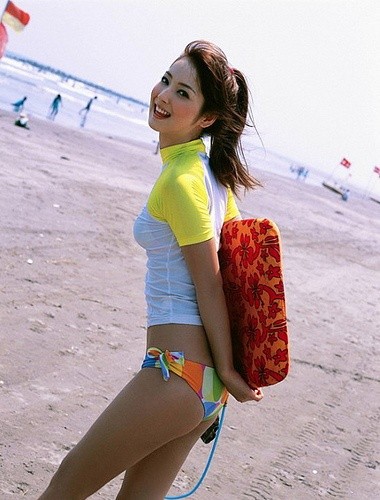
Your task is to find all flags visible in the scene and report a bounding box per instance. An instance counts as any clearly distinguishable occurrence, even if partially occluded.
[340,158,351,169]
[373,167,380,175]
[0,0,30,33]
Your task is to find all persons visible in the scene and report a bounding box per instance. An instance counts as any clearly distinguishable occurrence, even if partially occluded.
[36,41,264,500]
[14,96,27,107]
[340,189,350,200]
[49,94,63,112]
[80,96,98,113]
